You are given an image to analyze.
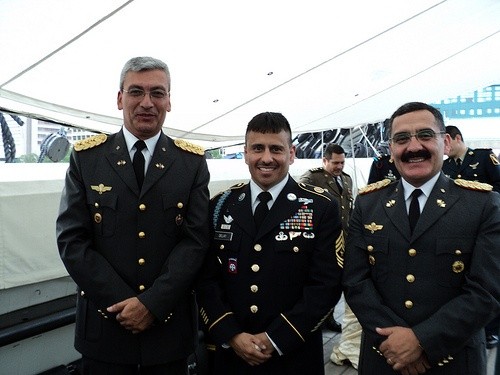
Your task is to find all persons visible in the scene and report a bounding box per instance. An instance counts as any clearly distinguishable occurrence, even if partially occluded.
[300,144,353,331]
[342,102,500,375]
[202,112,345,375]
[441,125,500,348]
[368,153,401,184]
[56,57,210,375]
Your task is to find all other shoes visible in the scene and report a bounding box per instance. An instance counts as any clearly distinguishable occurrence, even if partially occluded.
[324,320,341,332]
[487,333,498,346]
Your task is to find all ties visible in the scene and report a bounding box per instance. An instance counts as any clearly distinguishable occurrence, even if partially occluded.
[132,141,146,195]
[333,176,342,194]
[409,189,422,237]
[456,158,461,171]
[253,192,271,234]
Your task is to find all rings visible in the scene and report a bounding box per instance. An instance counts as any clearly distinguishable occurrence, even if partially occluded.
[388,358,394,365]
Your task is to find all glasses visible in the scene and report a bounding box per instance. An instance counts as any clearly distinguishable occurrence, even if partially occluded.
[389,130,445,147]
[122,89,170,100]
